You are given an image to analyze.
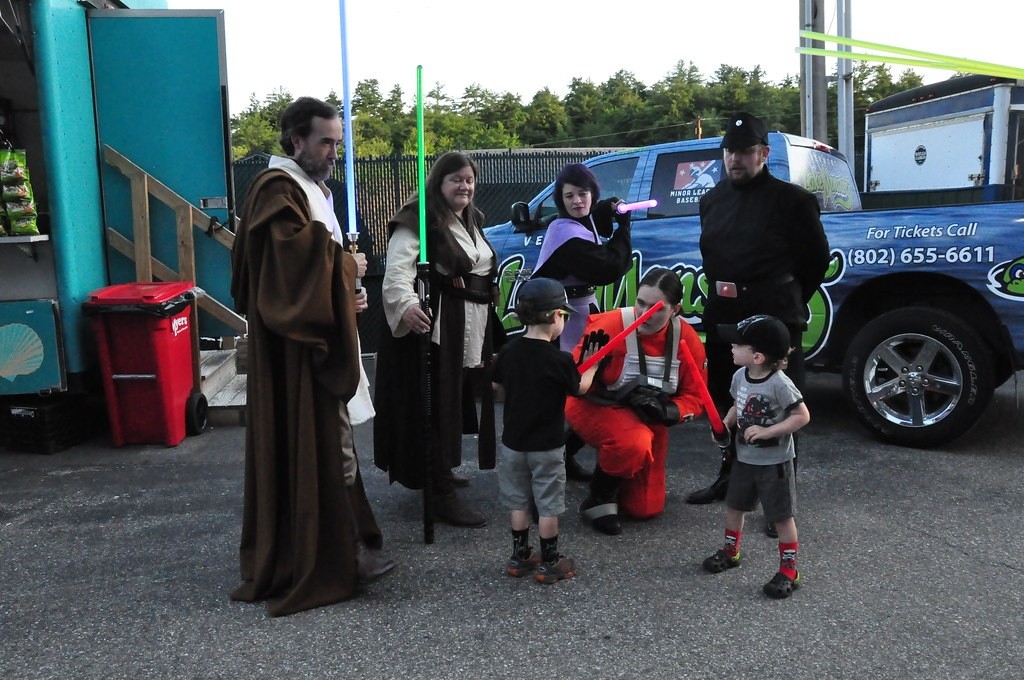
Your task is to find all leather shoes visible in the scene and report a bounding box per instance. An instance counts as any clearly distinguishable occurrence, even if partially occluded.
[353,539,400,587]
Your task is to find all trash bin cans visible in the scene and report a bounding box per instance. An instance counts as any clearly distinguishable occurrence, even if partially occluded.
[82,280,209,447]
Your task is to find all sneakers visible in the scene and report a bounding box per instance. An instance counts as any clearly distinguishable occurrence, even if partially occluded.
[506,545,542,577]
[535,555,579,584]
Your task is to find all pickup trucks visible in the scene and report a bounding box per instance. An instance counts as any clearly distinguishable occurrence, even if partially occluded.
[476,130,1024,447]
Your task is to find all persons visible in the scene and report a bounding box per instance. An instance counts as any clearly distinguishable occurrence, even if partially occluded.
[230,96,400,618]
[564,269,708,536]
[686,112,830,539]
[704,314,811,597]
[530,163,634,483]
[372,152,505,527]
[489,277,605,582]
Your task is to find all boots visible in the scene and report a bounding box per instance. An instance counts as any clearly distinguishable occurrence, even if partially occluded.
[423,468,487,528]
[763,508,776,536]
[563,427,590,482]
[450,468,470,486]
[686,446,733,505]
[586,461,627,535]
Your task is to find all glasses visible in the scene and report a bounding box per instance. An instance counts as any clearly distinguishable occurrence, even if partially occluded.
[543,307,569,324]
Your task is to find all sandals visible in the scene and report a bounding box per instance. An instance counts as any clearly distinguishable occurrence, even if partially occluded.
[702,549,743,573]
[764,567,800,600]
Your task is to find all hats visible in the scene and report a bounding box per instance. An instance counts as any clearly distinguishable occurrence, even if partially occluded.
[518,276,582,318]
[720,110,769,149]
[717,312,790,361]
